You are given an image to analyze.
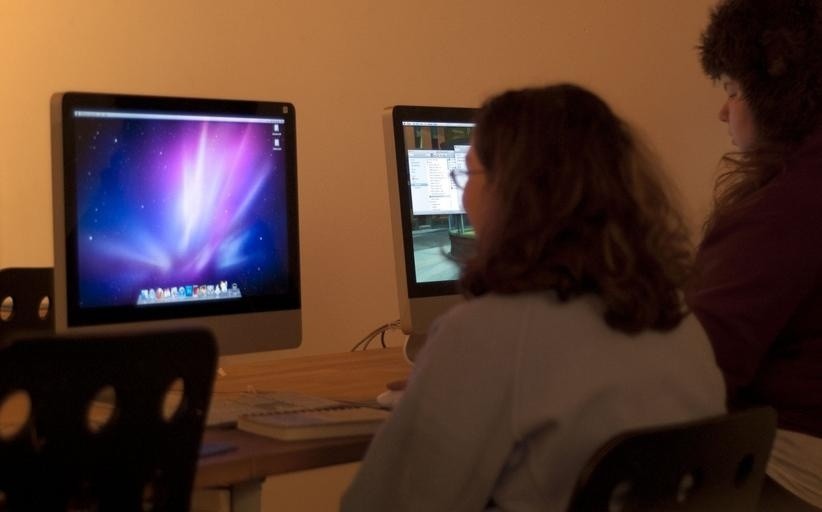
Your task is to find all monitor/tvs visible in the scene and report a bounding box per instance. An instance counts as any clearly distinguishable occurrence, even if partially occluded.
[47,90,303,358]
[380,104,480,338]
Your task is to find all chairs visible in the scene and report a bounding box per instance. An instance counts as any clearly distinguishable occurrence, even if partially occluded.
[566,406,779,512]
[0,267,54,338]
[0,327,219,512]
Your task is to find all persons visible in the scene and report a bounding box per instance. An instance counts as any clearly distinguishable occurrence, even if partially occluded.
[680,2,820,509]
[336,80,730,511]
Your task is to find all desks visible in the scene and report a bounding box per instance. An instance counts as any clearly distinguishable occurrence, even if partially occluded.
[142,348,411,512]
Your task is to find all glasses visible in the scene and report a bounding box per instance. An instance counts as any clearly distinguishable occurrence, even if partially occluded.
[450,163,486,189]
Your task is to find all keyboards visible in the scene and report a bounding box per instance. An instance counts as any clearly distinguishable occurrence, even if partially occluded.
[204,390,343,427]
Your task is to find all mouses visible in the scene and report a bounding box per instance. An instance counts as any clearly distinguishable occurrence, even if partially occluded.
[375,390,407,406]
